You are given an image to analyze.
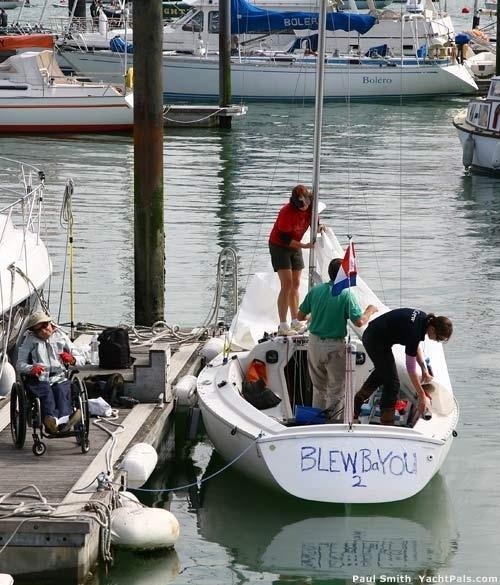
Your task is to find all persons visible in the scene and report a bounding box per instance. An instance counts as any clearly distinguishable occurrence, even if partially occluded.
[297,259,378,424]
[354,308,453,425]
[16,311,86,433]
[269,185,327,335]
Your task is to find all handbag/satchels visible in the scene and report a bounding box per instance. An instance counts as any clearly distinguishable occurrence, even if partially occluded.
[296,407,326,424]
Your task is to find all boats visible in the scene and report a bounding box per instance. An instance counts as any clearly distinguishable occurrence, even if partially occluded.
[196,220,462,511]
[0,49,136,139]
[450,76,500,179]
[192,463,463,584]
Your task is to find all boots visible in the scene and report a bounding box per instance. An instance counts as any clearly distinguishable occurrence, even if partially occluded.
[355,386,370,416]
[380,407,395,425]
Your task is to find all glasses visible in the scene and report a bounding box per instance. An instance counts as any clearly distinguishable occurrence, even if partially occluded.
[35,323,48,332]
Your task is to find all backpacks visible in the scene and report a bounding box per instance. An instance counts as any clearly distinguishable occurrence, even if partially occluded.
[242,359,282,410]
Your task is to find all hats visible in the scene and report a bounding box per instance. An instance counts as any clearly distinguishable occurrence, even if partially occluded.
[461,7,470,13]
[25,311,52,330]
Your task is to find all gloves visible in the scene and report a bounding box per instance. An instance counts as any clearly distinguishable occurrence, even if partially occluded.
[32,365,45,375]
[59,352,73,363]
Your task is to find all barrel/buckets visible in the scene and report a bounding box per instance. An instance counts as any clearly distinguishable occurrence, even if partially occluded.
[121,441,158,491]
[295,404,327,425]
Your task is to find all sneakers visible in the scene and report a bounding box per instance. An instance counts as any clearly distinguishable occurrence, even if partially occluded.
[44,409,81,434]
[277,322,304,336]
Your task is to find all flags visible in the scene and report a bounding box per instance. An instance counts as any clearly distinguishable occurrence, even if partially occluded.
[331,240,357,296]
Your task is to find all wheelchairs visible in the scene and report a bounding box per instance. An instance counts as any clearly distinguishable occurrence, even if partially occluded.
[9,349,91,456]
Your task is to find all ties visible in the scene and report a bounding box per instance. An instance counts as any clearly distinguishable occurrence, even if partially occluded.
[44,339,61,377]
